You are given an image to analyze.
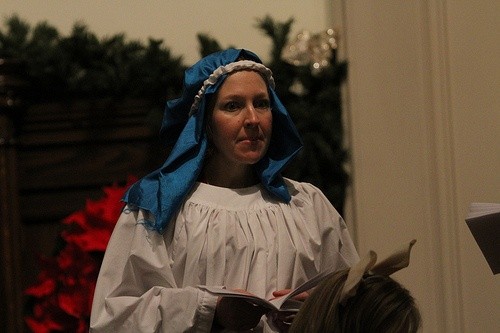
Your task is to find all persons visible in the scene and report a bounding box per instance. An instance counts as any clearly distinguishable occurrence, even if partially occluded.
[288,239,422,333]
[90,49,360,333]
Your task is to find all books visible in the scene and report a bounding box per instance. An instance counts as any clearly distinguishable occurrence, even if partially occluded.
[195,270,333,317]
[465,202,500,275]
